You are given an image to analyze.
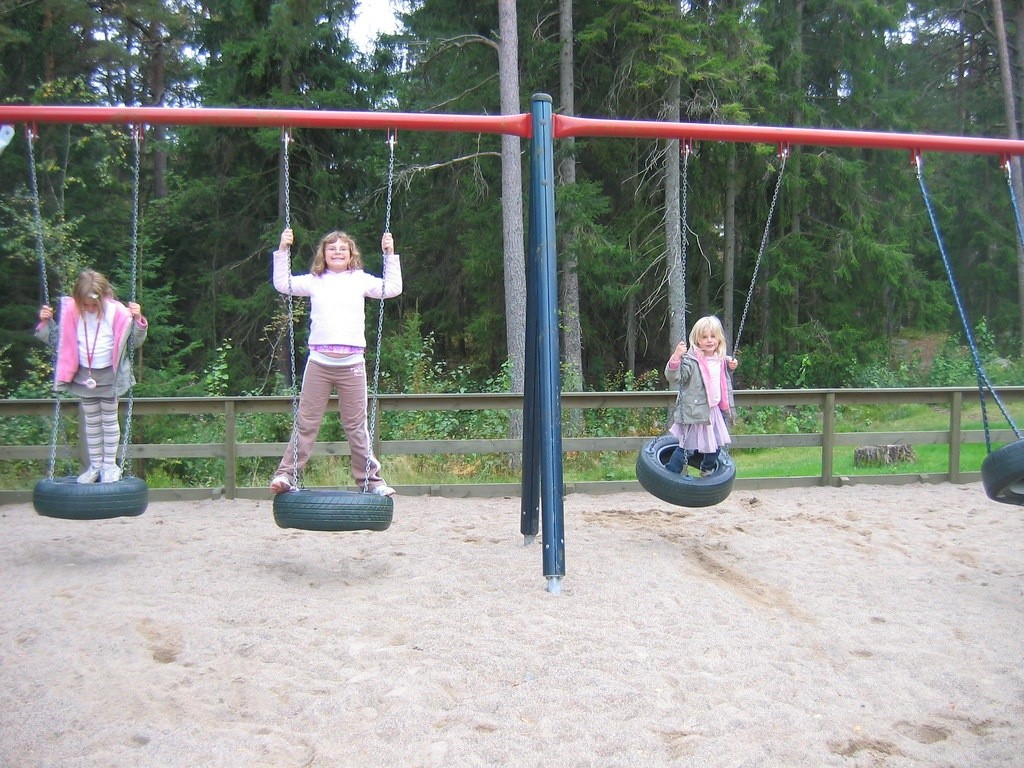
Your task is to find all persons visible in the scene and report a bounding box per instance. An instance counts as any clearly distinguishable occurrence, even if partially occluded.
[34,268,148,484]
[664,315,739,478]
[271,227,403,496]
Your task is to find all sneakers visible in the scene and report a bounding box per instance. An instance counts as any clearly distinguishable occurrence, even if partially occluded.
[270,477,299,493]
[366,484,396,497]
[77,463,122,483]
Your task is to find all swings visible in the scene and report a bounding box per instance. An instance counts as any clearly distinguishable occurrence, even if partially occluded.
[907,153,1024,509]
[23,119,152,522]
[634,148,791,510]
[270,131,400,533]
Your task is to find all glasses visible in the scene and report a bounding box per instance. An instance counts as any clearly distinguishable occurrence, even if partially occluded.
[325,246,350,254]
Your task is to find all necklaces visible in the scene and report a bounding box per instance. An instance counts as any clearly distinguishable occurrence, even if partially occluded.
[80,309,103,390]
